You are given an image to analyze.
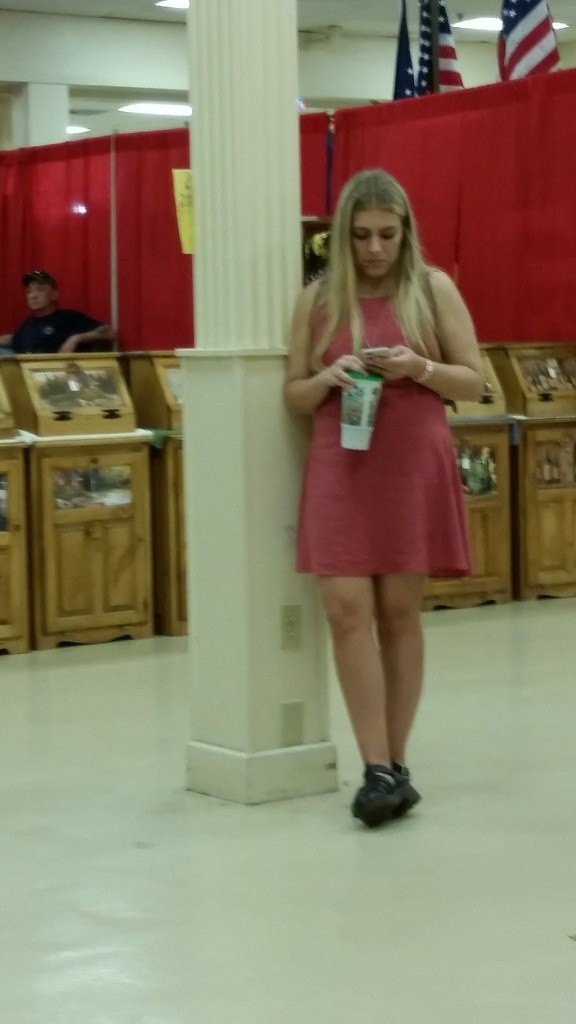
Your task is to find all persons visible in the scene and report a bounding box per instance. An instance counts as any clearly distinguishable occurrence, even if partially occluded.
[279,166,490,831]
[0,268,116,354]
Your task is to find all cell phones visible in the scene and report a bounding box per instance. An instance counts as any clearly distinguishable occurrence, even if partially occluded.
[361,347,391,365]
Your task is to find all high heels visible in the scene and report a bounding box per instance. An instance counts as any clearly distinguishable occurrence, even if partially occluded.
[350,761,420,826]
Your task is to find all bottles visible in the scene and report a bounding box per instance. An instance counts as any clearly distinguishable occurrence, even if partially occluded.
[543,451,553,484]
[553,458,560,483]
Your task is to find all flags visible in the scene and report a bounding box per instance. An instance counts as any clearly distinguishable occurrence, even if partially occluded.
[496,0,560,79]
[390,0,466,106]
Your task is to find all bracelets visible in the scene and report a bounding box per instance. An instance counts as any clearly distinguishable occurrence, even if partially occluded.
[413,358,434,384]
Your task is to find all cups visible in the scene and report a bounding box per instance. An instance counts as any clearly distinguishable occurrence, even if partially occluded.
[340,373,383,451]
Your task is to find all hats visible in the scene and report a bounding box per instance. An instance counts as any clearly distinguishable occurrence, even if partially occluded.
[22,270,55,289]
